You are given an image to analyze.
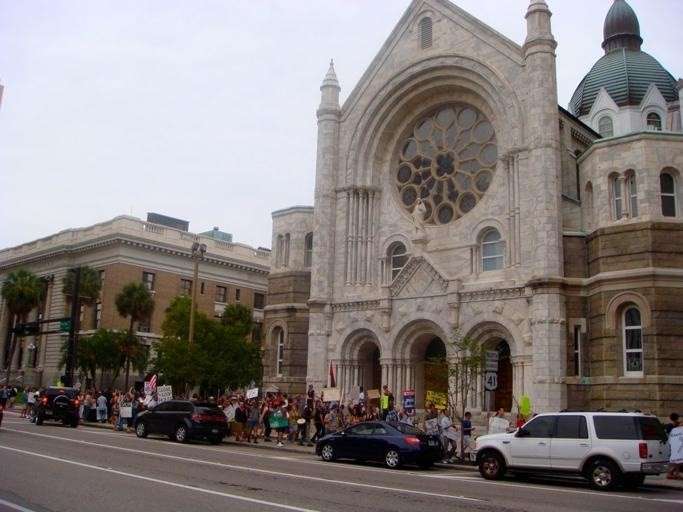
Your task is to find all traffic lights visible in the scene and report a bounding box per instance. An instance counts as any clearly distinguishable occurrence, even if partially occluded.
[14,322,39,337]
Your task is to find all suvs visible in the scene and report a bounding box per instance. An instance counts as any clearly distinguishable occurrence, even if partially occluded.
[471,410,675,492]
[30,383,83,429]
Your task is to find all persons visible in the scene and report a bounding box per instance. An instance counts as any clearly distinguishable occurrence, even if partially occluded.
[515,405,525,428]
[666,412,681,479]
[488,407,512,436]
[664,414,683,480]
[56,376,65,388]
[0,383,39,419]
[74,383,474,466]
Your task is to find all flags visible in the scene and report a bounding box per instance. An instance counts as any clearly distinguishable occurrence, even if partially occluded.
[145,372,156,393]
[325,360,334,387]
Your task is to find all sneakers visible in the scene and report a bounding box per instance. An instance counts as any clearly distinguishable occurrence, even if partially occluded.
[19,415,30,419]
[442,456,459,465]
[113,427,132,433]
[235,434,316,448]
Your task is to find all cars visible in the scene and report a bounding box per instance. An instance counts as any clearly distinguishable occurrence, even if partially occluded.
[131,399,230,446]
[313,420,450,472]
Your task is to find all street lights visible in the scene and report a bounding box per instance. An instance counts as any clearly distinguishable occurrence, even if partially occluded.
[26,342,36,367]
[187,239,209,343]
